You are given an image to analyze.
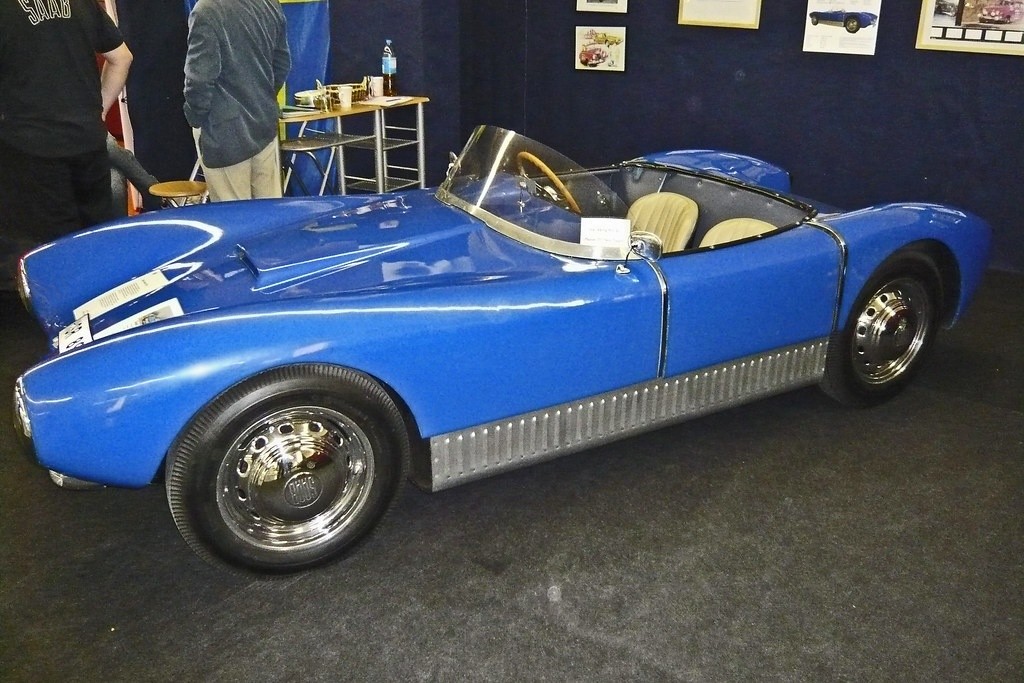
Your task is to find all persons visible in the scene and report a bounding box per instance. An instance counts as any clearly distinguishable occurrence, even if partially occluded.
[0,0,134,247]
[182,0,291,204]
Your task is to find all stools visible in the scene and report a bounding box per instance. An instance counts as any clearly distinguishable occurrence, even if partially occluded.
[149,181,207,209]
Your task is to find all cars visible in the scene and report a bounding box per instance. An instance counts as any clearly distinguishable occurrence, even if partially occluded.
[579,32,623,67]
[979,6,1014,24]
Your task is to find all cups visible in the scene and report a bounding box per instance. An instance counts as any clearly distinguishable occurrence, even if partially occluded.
[338,86,353,109]
[368,76,384,97]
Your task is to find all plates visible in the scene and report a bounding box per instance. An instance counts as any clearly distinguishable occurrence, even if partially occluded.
[294,89,320,108]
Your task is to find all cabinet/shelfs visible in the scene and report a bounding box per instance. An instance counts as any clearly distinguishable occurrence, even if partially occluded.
[279,94,429,196]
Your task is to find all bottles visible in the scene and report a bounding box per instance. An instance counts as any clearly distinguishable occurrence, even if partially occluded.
[382,38,398,97]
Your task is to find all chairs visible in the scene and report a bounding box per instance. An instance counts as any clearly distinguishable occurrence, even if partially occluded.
[626,192,699,253]
[699,217,777,247]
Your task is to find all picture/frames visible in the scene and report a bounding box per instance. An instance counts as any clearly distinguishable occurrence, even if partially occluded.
[915,0,1024,56]
[678,0,761,29]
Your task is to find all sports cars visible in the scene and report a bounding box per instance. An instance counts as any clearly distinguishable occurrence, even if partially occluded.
[10,123,996,580]
[809,9,878,34]
